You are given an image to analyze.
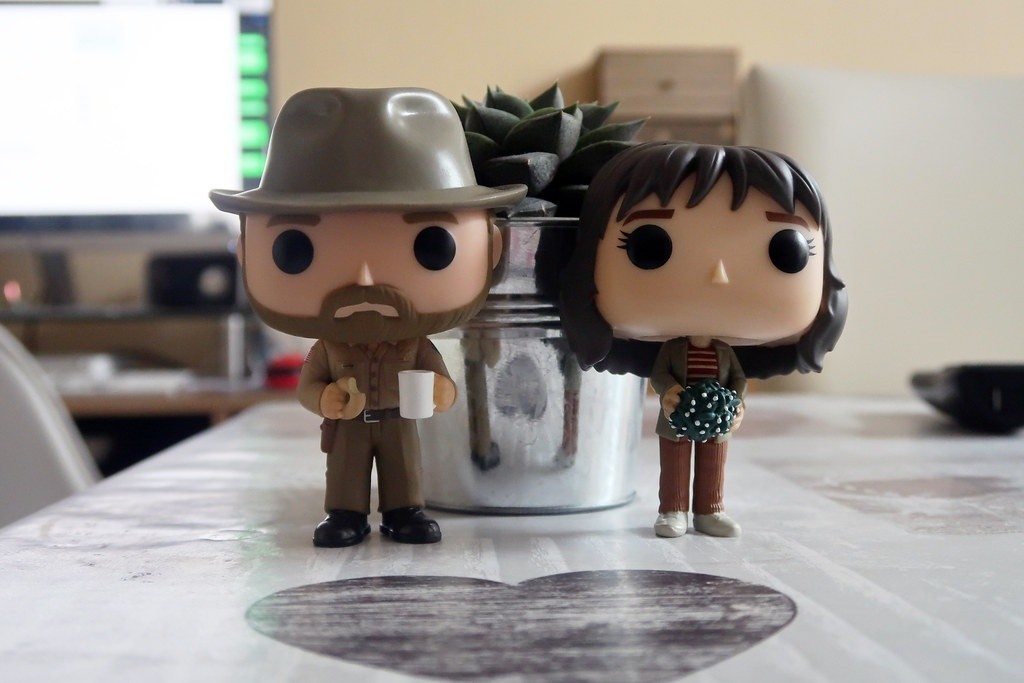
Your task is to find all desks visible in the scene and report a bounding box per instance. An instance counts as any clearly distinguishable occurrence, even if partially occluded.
[0,309,291,429]
[0,398,1024,683]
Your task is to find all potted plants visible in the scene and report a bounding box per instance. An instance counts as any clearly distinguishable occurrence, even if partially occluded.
[414,81,649,516]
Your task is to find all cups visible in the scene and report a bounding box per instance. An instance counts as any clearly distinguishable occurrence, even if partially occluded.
[398,370,436,419]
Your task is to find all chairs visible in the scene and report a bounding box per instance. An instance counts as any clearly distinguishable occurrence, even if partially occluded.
[142,250,238,315]
[0,325,106,529]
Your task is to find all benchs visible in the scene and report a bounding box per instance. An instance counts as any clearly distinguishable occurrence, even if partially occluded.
[50,381,265,427]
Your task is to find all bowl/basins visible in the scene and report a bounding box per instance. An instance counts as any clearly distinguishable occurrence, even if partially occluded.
[910,364,1024,436]
